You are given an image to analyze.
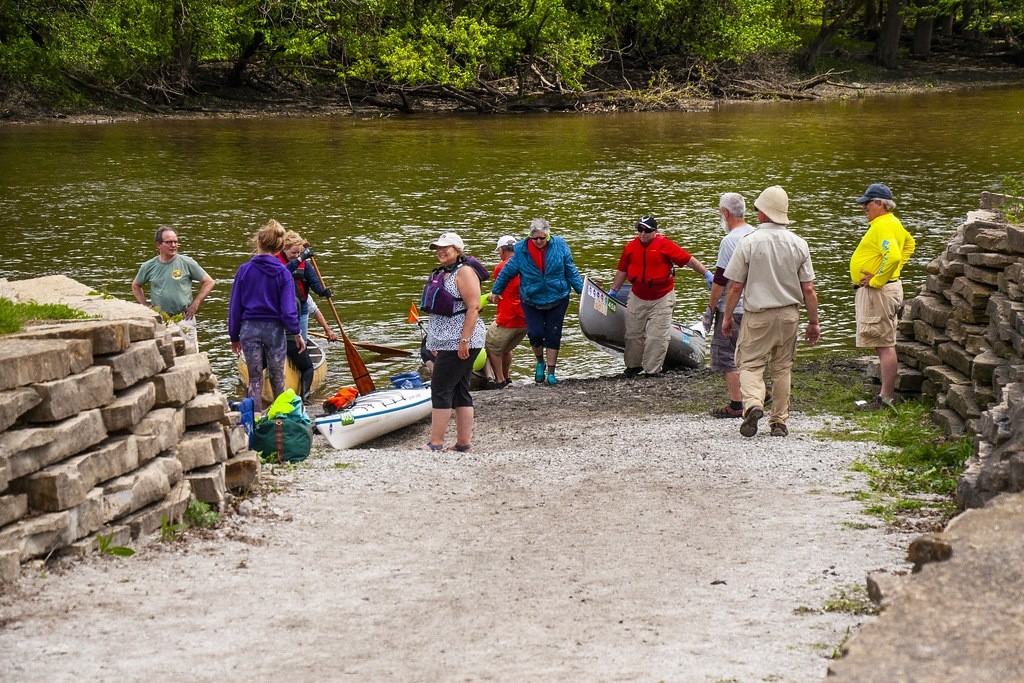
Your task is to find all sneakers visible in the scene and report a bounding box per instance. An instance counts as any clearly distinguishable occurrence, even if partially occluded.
[770,422,788,436]
[485,377,513,390]
[709,404,743,419]
[740,407,763,437]
[856,392,896,412]
[763,391,772,407]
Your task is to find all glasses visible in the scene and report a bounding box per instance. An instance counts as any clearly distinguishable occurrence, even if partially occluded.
[755,207,758,211]
[159,241,178,245]
[532,236,546,240]
[861,200,874,205]
[638,226,655,233]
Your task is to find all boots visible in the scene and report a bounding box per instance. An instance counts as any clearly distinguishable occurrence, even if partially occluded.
[300,381,313,406]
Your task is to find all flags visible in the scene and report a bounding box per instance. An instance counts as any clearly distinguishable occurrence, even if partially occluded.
[407,303,419,322]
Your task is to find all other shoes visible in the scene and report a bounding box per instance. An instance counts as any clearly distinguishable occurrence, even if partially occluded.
[535,361,558,385]
[620,366,658,378]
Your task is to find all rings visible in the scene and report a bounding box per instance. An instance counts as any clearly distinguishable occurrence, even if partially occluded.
[463,354,465,355]
[815,338,817,339]
[189,314,191,316]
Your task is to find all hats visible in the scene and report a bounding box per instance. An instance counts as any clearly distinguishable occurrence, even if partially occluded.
[639,215,657,230]
[429,233,464,250]
[856,183,894,203]
[754,185,790,224]
[494,236,517,252]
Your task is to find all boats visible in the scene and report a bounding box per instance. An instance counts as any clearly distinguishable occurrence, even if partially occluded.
[315,373,434,450]
[579,275,707,369]
[238,333,327,404]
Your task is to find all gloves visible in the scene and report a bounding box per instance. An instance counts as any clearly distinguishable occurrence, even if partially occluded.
[703,304,715,333]
[703,270,714,290]
[298,248,314,261]
[322,288,331,298]
[608,288,618,298]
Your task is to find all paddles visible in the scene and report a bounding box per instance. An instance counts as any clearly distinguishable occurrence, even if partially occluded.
[307,331,414,357]
[408,302,429,334]
[303,242,375,395]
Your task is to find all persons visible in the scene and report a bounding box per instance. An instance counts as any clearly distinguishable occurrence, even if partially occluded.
[132,225,215,355]
[721,186,820,436]
[491,218,585,384]
[421,292,503,375]
[408,233,486,453]
[246,230,337,405]
[703,193,773,419]
[228,220,306,417]
[607,215,715,379]
[485,235,527,389]
[850,184,915,412]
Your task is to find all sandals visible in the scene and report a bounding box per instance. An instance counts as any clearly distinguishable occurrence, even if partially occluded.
[416,441,443,452]
[447,443,473,453]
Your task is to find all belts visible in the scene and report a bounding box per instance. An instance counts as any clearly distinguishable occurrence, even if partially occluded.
[854,280,898,290]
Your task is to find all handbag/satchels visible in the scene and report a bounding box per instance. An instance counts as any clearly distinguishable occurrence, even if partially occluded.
[391,371,423,389]
[229,395,313,464]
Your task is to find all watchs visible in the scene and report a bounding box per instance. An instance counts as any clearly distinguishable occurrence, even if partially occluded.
[462,338,470,344]
